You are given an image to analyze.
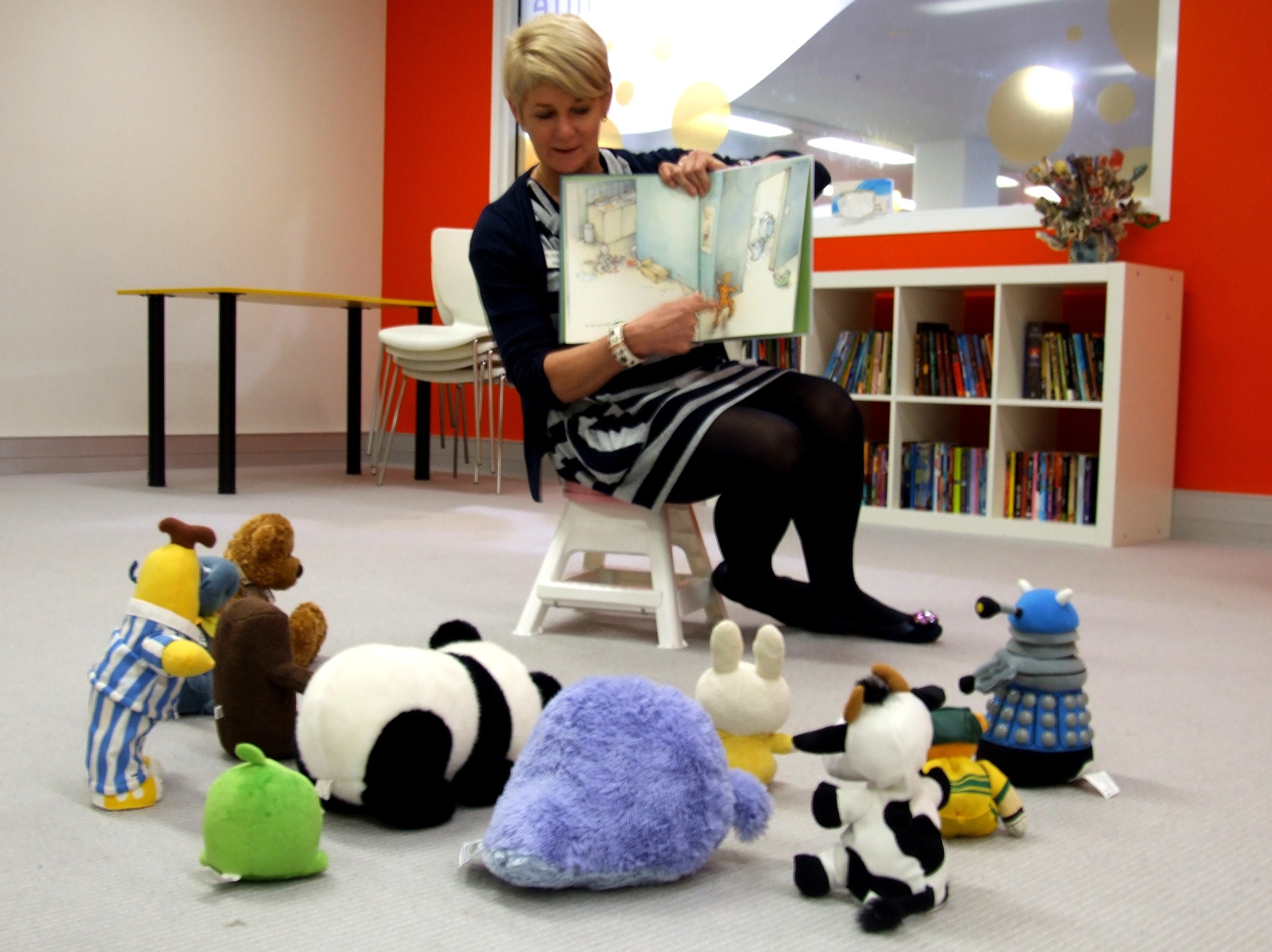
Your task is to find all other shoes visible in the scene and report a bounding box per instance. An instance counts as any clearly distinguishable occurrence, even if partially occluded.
[712,565,809,624]
[803,600,941,643]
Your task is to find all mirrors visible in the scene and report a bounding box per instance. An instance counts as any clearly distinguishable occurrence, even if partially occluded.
[491,0,1179,238]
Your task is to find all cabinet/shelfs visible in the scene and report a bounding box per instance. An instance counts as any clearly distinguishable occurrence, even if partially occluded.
[725,261,1185,547]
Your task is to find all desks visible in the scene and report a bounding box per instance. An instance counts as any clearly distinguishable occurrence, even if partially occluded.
[115,286,439,495]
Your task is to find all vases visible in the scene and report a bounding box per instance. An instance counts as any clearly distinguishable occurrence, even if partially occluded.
[1069,230,1117,263]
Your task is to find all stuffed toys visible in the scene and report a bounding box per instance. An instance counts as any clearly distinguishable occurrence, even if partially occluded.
[918,706,1025,838]
[222,512,328,668]
[211,598,314,758]
[481,674,774,888]
[175,555,240,715]
[297,620,563,828]
[199,743,328,880]
[695,620,796,783]
[85,517,216,810]
[791,663,950,932]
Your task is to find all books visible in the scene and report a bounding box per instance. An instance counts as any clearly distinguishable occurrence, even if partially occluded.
[1003,449,1099,524]
[901,440,988,515]
[560,155,814,344]
[745,336,801,370]
[861,440,889,506]
[824,331,892,394]
[916,322,993,398]
[1023,322,1104,401]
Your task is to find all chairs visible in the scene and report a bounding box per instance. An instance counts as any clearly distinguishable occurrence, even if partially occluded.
[366,227,514,495]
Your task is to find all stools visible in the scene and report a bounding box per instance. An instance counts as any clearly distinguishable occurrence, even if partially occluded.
[512,481,730,649]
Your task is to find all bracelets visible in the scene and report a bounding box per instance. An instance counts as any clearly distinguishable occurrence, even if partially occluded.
[608,322,647,368]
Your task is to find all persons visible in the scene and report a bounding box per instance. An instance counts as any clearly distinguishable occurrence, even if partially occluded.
[468,15,943,643]
